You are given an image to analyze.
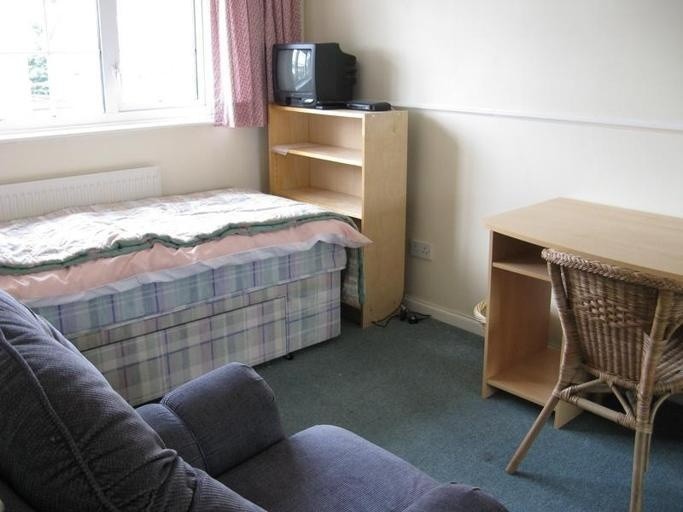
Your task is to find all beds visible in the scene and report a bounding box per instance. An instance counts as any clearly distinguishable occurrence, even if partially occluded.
[0,186,375,407]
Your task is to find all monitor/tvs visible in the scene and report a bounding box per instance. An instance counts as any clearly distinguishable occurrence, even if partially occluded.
[271,42,357,110]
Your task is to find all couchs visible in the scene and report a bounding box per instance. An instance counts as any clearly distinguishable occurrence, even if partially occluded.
[0,287,509,511]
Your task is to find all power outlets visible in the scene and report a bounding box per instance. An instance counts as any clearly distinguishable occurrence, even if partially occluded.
[410,239,431,261]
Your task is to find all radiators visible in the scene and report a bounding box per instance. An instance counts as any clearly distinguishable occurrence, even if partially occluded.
[1,166,161,222]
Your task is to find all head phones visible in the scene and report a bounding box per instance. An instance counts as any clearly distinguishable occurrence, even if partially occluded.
[399,306,431,323]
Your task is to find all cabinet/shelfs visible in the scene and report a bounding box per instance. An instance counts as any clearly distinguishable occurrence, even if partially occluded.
[267,106,408,330]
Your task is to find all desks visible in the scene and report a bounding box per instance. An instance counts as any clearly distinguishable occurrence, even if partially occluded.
[481,197,683,429]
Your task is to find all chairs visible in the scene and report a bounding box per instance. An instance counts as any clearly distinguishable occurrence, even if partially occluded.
[505,248,683,512]
